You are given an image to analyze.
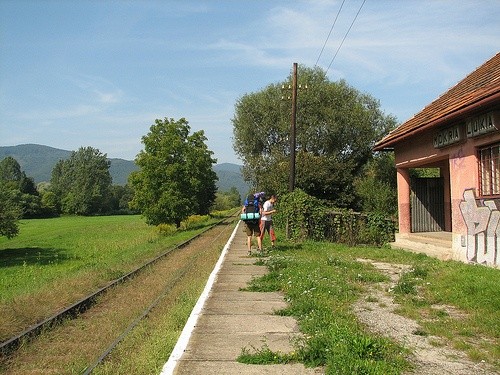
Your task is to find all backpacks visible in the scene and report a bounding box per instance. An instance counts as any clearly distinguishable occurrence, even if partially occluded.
[244,200,259,227]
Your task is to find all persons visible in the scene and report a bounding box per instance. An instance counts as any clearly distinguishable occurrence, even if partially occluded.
[257,193,277,251]
[253,191,269,208]
[242,194,264,256]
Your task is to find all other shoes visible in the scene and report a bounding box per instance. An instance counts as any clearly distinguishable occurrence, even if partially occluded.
[271,245,275,249]
[257,249,263,255]
[247,252,251,256]
